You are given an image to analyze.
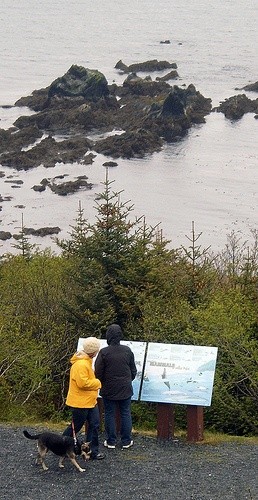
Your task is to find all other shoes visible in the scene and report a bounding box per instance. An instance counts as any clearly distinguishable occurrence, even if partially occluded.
[92,453,106,460]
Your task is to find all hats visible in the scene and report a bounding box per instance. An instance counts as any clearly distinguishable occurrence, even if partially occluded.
[82,337,100,353]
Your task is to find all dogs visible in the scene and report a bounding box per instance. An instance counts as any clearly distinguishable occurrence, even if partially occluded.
[23,430,93,472]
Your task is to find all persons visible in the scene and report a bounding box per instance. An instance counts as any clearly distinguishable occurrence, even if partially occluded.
[95,324,137,449]
[62,337,103,459]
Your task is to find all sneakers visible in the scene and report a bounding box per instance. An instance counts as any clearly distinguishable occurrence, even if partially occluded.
[104,440,115,449]
[122,440,133,448]
